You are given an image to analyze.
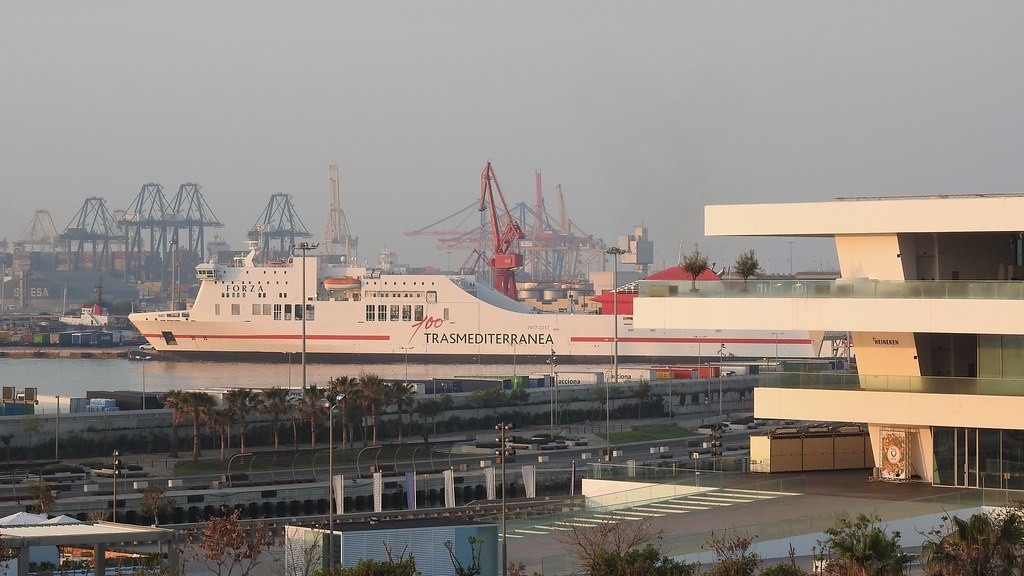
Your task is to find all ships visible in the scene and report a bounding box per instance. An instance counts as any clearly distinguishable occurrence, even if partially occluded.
[127,224,855,361]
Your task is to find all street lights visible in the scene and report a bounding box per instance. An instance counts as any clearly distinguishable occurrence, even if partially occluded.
[401,346,413,392]
[169,239,175,311]
[280,351,297,404]
[113,450,121,524]
[168,239,176,311]
[289,241,319,401]
[427,377,439,439]
[773,332,783,372]
[319,376,346,576]
[495,422,516,576]
[717,343,726,430]
[55,394,59,464]
[604,337,619,462]
[605,247,627,384]
[695,335,706,379]
[666,366,673,425]
[135,356,152,413]
[603,246,626,383]
[546,348,559,441]
[789,241,792,277]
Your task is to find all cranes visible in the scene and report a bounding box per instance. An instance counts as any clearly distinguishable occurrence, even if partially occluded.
[477,160,524,301]
